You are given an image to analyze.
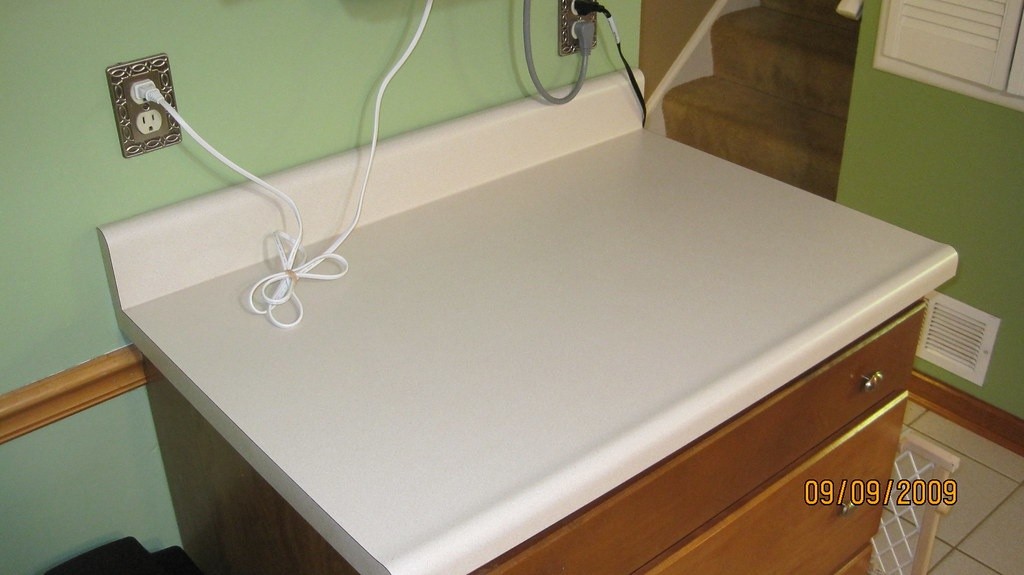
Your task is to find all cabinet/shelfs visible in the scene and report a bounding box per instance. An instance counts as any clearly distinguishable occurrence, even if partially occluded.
[97,65,960,575]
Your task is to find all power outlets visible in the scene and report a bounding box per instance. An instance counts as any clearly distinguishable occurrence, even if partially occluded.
[558,0,598,57]
[105,52,183,158]
